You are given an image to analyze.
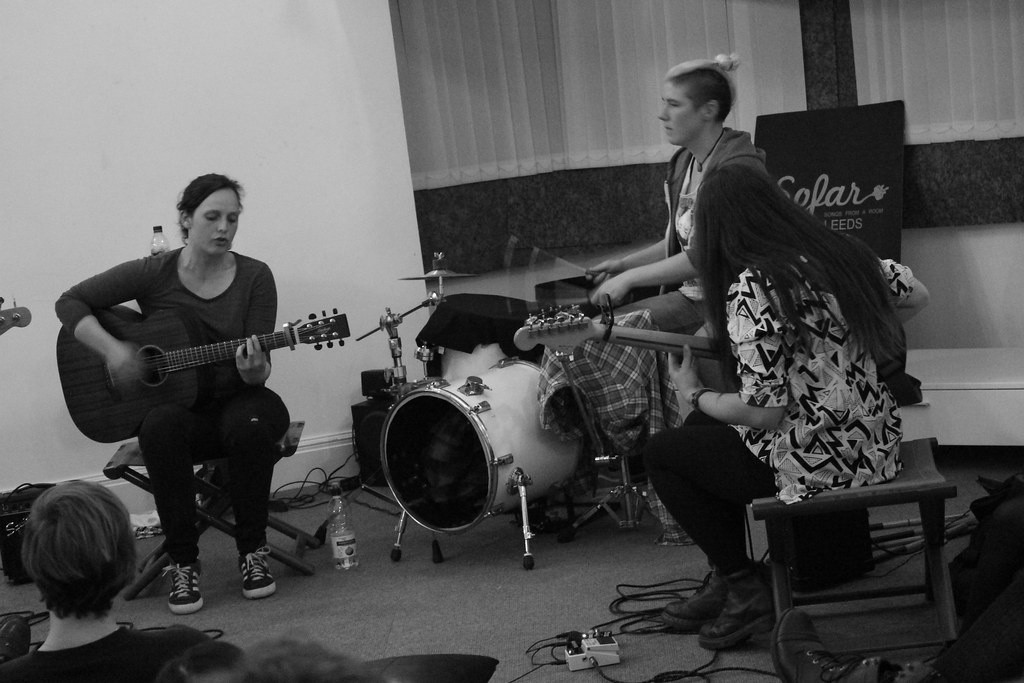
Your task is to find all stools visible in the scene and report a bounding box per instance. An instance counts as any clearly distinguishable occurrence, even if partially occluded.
[752,436,959,643]
[104,422,324,603]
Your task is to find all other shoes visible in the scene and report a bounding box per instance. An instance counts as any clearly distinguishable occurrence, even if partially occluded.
[0,617,31,663]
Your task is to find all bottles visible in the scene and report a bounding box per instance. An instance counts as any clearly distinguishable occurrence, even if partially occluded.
[151,226,170,256]
[328,482,359,570]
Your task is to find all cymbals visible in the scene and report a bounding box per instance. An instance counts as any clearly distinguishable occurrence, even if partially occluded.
[398,268,478,281]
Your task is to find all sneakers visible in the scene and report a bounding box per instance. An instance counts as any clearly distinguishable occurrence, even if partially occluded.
[699,566,775,650]
[661,566,748,633]
[163,559,203,615]
[237,546,277,599]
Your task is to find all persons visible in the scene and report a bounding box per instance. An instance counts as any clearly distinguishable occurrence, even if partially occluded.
[583,51,768,333]
[640,162,931,652]
[55,173,290,614]
[0,479,212,683]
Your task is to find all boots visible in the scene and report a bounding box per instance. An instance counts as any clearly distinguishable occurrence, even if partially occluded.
[770,607,888,683]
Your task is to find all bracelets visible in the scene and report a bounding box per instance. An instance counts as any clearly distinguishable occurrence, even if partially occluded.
[692,387,718,412]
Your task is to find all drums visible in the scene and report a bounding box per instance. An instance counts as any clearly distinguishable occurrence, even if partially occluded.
[435,294,550,386]
[378,357,583,536]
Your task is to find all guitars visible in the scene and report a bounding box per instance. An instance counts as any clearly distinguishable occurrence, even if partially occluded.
[514,302,909,393]
[0,297,32,339]
[54,306,352,445]
